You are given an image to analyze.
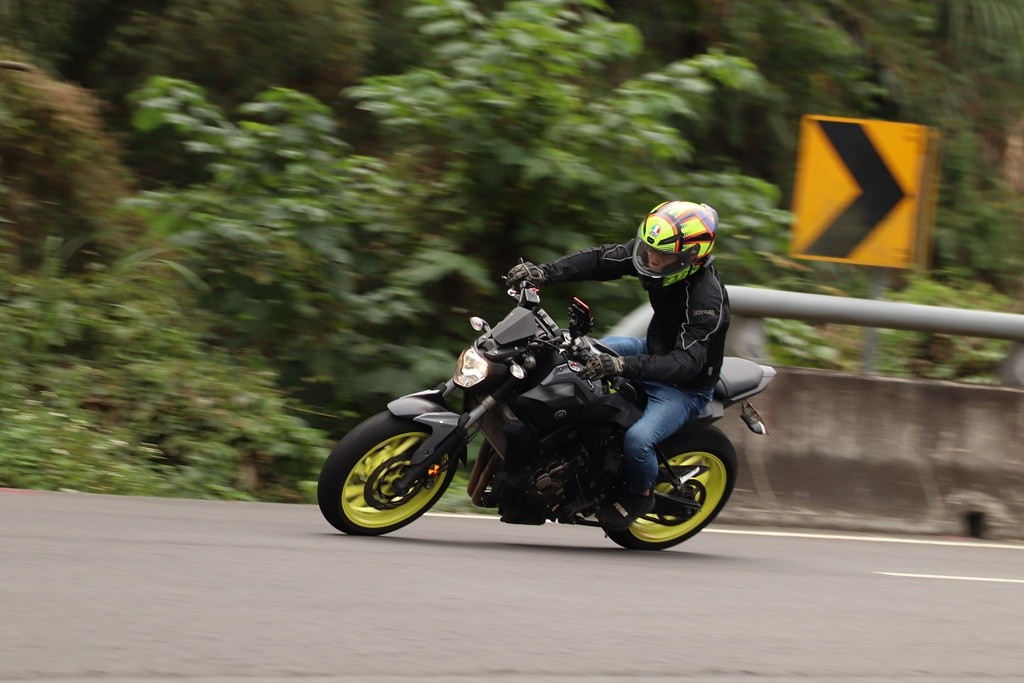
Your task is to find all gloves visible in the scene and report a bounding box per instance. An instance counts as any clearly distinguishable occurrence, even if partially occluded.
[506,261,550,290]
[577,352,624,382]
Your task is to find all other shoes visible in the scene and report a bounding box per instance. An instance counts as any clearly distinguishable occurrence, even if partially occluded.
[599,481,655,531]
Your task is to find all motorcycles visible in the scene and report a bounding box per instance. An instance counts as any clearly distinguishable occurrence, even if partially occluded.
[315,257,776,550]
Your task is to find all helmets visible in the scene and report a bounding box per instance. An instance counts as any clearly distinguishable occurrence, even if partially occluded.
[631,200,719,288]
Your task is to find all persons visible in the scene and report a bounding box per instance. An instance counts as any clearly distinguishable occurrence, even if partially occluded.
[505,199,731,531]
